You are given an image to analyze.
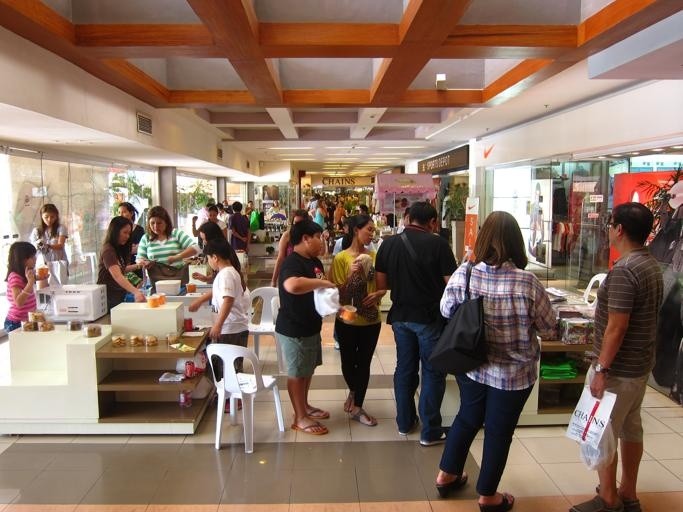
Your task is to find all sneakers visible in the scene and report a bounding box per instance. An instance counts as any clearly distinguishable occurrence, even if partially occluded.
[569,485,640,512]
[419,427,451,446]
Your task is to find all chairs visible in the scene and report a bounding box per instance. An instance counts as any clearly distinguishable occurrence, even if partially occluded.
[205,343,285,453]
[583,273,609,308]
[246,286,286,373]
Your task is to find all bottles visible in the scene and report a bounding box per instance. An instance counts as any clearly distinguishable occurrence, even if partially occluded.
[367,271,377,293]
[314,267,324,280]
[259,223,286,243]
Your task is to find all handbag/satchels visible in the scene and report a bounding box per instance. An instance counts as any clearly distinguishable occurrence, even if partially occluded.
[429,296,486,374]
[147,262,189,285]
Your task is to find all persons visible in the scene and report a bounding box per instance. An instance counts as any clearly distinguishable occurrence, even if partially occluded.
[30,204,70,286]
[168,193,410,436]
[3,241,37,333]
[375,201,458,447]
[568,201,665,511]
[436,210,557,512]
[135,205,196,288]
[118,202,145,279]
[97,216,147,314]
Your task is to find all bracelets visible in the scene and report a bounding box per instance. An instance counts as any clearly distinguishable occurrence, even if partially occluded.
[22,290,31,295]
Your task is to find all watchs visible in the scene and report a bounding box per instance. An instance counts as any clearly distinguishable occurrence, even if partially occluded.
[594,362,610,373]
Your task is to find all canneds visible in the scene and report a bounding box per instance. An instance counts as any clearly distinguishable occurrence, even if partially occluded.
[184,361,196,379]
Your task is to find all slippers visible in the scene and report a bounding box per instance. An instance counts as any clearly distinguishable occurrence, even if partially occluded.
[291,408,329,435]
[437,473,467,497]
[344,392,377,425]
[479,494,514,512]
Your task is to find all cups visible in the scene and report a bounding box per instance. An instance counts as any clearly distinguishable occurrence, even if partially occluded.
[38,267,48,278]
[343,305,357,323]
[146,292,166,307]
[179,391,193,408]
[167,332,178,348]
[185,361,196,378]
[184,318,193,331]
[186,284,197,293]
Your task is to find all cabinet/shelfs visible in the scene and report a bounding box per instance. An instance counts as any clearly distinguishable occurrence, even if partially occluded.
[0,302,215,435]
[516,336,593,426]
[254,183,290,220]
[249,231,281,257]
